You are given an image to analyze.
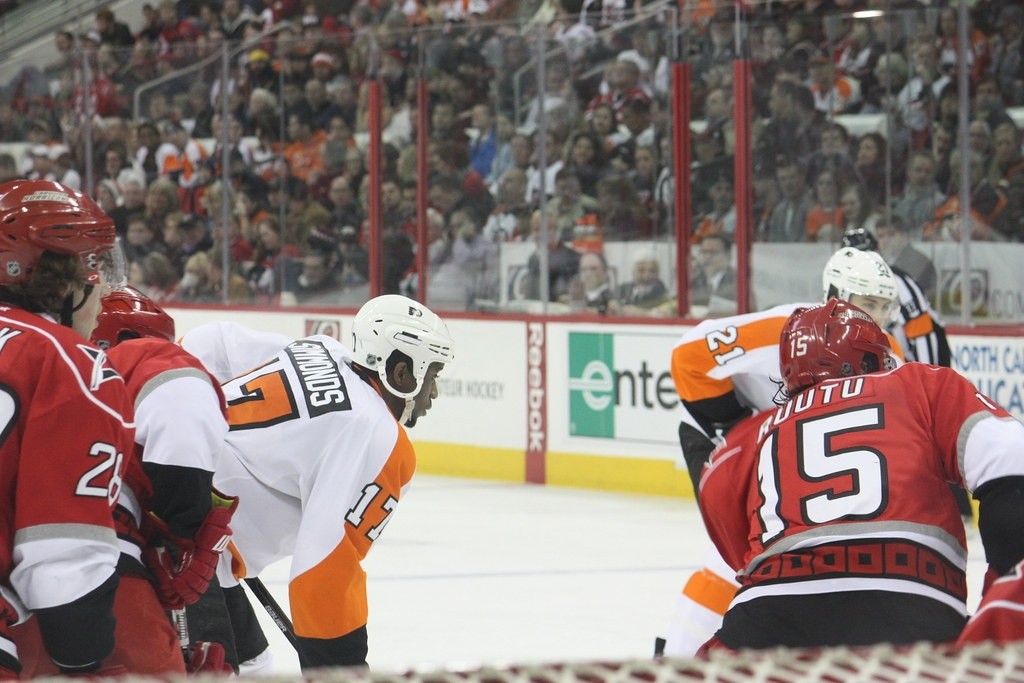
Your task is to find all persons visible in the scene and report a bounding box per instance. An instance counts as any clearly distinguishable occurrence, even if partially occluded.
[669,247,896,648]
[0,1,1024,312]
[697,300,1024,649]
[0,178,457,683]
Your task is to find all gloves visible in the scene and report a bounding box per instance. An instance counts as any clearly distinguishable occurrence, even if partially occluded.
[183,640,236,680]
[139,485,240,605]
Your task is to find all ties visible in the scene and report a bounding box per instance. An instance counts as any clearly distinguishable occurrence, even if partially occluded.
[475,131,485,150]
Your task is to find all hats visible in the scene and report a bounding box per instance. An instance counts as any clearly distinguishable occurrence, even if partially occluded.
[177,214,203,228]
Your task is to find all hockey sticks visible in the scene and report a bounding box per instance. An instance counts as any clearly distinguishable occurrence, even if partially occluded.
[245,577,297,650]
[172,604,190,673]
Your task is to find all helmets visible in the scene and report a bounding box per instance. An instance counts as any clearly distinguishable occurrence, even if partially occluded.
[822,247,897,306]
[0,179,125,284]
[350,294,456,402]
[840,229,880,251]
[779,299,896,394]
[92,286,176,348]
